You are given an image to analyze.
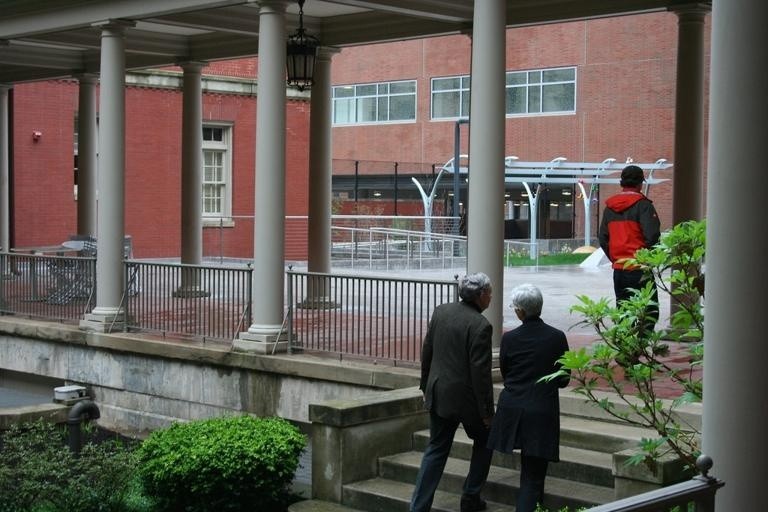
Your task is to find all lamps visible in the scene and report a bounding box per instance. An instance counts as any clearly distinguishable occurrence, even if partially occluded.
[284,0,321,90]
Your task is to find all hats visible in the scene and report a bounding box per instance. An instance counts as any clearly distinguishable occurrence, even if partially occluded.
[621,165,647,184]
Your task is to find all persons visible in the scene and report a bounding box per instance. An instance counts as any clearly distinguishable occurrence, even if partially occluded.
[599,166,662,378]
[488,283,571,512]
[409,272,495,512]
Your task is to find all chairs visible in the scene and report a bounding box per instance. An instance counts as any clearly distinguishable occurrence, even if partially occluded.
[48,235,131,307]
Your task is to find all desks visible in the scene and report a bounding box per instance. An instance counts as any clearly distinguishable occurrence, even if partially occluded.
[13,245,77,304]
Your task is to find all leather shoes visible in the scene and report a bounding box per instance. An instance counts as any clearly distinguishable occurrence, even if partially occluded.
[460,498,488,512]
